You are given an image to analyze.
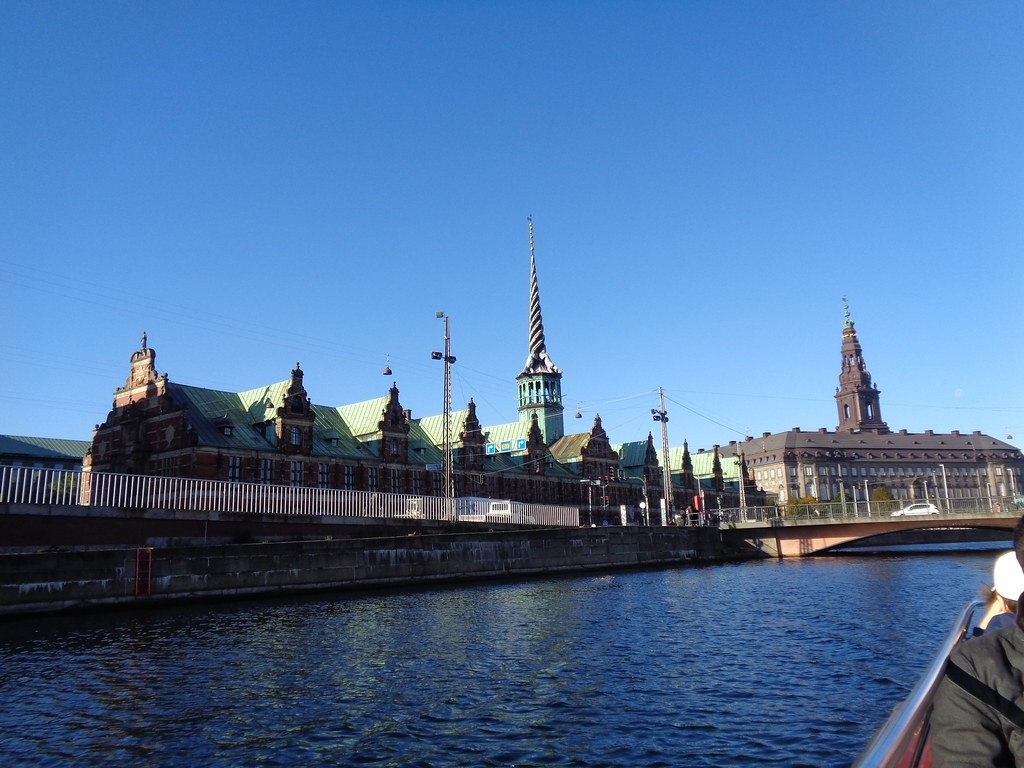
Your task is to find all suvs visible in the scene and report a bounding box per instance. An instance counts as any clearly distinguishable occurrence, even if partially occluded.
[890,503,940,516]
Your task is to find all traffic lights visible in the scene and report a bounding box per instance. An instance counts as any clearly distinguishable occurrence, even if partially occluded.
[608,465,615,482]
[604,476,609,486]
[599,495,609,508]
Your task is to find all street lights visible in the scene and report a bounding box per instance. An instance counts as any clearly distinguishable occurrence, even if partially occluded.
[864,479,872,517]
[629,476,648,524]
[922,480,930,503]
[851,485,858,516]
[813,475,819,502]
[1006,468,1016,500]
[938,463,950,514]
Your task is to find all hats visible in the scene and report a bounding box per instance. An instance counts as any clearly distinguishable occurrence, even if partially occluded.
[991,551,1022,600]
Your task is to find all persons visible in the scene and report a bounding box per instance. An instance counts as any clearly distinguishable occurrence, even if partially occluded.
[930,512,1024,768]
